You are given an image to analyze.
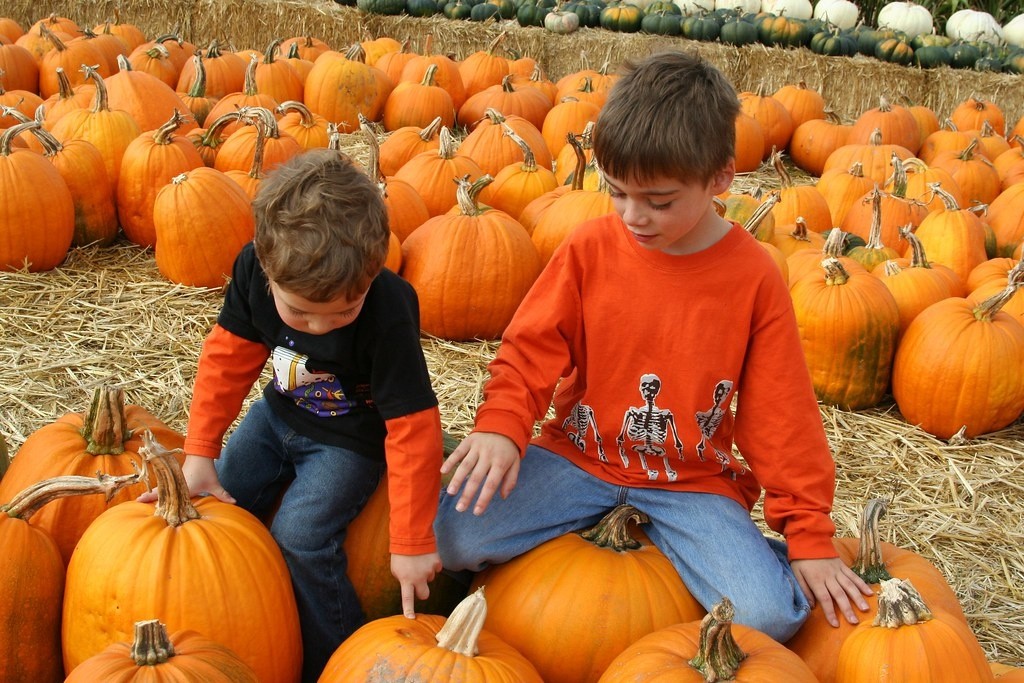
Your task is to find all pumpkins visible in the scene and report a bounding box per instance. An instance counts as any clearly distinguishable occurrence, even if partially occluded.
[0,0,1024,683]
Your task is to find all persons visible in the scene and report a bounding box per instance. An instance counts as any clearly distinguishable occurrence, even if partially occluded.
[428,46,873,649]
[134,145,445,683]
